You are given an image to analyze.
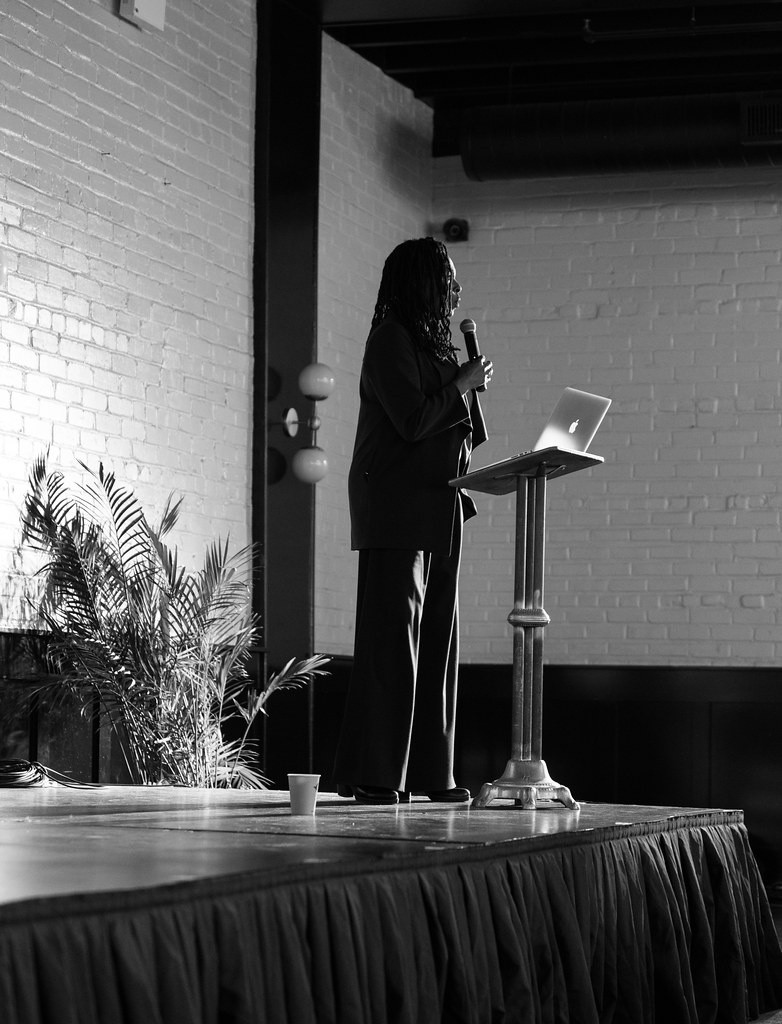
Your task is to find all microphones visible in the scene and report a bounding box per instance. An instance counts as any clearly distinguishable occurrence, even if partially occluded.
[458,318,489,393]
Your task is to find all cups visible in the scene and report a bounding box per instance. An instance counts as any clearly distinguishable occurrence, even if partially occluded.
[287,773,321,816]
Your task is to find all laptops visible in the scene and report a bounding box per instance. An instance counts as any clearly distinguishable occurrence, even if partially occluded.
[469,386,612,475]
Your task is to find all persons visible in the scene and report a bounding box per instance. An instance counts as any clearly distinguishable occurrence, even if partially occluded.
[333,236,493,805]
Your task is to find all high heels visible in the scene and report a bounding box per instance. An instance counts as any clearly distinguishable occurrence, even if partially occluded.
[399,788,470,803]
[335,779,397,805]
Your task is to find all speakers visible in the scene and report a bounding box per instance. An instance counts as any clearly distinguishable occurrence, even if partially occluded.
[119,0,166,31]
